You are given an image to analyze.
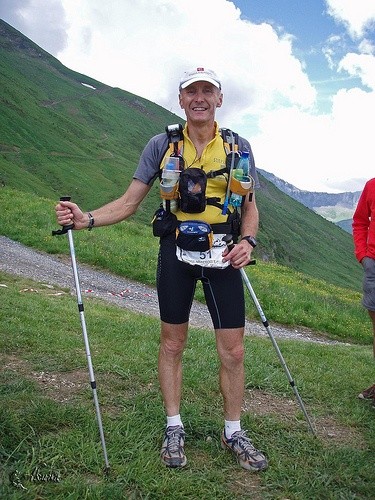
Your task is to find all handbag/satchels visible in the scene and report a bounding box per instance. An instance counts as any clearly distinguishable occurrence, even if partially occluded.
[175,220,214,252]
[179,168,207,213]
[151,207,169,237]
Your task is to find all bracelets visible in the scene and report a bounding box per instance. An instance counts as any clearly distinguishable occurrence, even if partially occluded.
[87,211,93,232]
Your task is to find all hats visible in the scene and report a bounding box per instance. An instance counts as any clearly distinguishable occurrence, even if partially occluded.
[180,66,221,89]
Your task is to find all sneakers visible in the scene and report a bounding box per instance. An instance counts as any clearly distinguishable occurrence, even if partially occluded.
[220,428,268,471]
[160,425,187,469]
[358,384,375,400]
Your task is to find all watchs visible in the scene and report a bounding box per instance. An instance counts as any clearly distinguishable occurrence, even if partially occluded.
[239,236,259,248]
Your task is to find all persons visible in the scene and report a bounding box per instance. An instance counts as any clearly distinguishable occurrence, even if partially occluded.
[54,66,267,473]
[350,163,375,402]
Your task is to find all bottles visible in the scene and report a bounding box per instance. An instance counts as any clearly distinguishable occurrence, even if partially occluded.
[230,151,249,207]
[163,157,179,192]
